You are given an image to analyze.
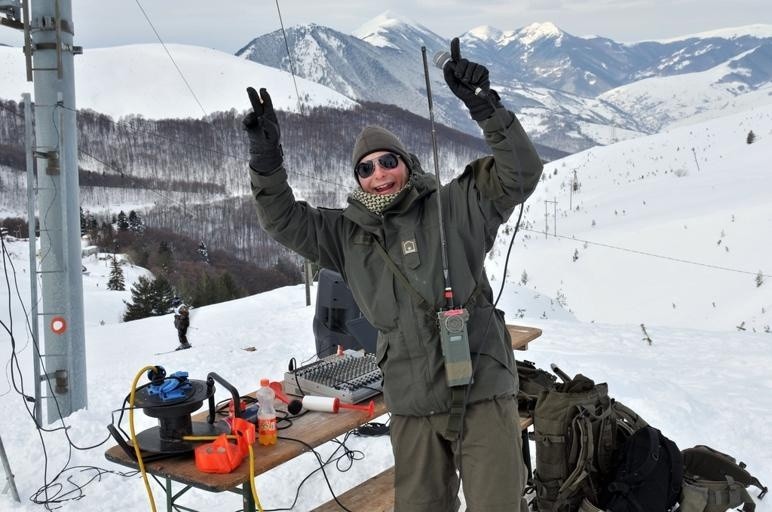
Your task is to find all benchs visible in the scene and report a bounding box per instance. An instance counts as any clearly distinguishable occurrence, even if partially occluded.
[308,416,534,511]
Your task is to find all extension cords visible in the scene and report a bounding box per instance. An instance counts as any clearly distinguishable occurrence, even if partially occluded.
[359,426,390,436]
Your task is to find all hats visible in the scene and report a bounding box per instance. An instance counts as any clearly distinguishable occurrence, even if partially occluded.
[172,297,180,304]
[353,125,413,180]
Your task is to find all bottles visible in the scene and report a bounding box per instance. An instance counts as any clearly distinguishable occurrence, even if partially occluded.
[257,379,278,445]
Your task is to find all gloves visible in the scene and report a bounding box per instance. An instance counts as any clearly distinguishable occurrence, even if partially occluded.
[242,86,283,174]
[444,37,500,121]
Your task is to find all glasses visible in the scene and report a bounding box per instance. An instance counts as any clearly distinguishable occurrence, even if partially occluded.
[355,152,401,178]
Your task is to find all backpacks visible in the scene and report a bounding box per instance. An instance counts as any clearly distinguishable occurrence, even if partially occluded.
[517,361,768,512]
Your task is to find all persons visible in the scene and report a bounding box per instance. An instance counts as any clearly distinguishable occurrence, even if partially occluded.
[244,37,545,511]
[169,296,192,352]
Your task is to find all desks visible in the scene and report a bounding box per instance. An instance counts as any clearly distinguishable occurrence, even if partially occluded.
[104,324,543,512]
